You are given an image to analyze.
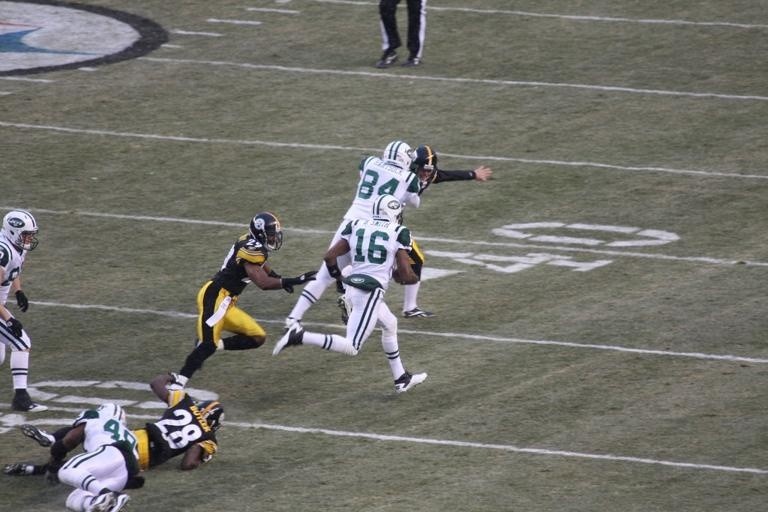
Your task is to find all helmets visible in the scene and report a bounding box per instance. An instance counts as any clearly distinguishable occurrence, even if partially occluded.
[249,211,283,251]
[401,307,437,318]
[96,403,127,424]
[2,210,38,251]
[373,195,403,222]
[413,144,437,179]
[197,401,224,430]
[383,141,412,169]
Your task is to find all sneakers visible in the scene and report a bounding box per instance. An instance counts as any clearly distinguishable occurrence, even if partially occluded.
[88,492,118,512]
[395,372,427,394]
[4,463,33,475]
[337,295,352,324]
[21,424,52,447]
[402,57,421,66]
[12,399,47,412]
[273,321,304,356]
[109,494,128,512]
[377,52,398,67]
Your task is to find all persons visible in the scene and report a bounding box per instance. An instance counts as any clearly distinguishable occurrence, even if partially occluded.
[1,373,225,480]
[377,0,430,70]
[336,143,494,326]
[56,400,139,510]
[274,192,429,397]
[168,212,316,387]
[283,136,440,331]
[1,207,48,416]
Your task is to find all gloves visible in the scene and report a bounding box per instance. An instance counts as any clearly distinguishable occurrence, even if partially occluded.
[15,290,28,311]
[46,464,59,484]
[6,317,22,338]
[282,271,318,287]
[169,372,178,382]
